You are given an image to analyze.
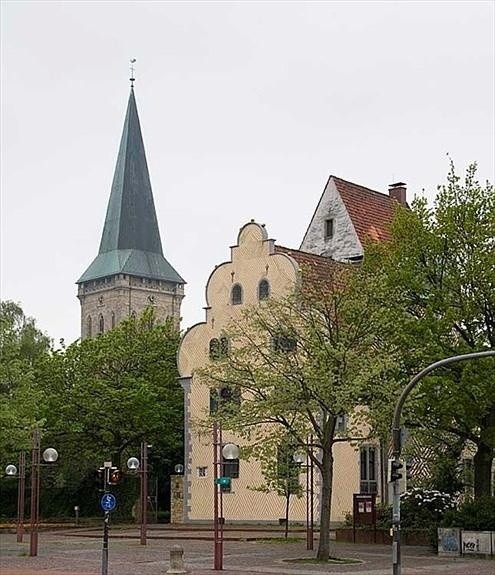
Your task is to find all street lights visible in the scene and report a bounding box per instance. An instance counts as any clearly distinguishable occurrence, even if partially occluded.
[212,420,241,570]
[126,442,149,546]
[293,435,314,550]
[29,430,58,555]
[4,450,26,544]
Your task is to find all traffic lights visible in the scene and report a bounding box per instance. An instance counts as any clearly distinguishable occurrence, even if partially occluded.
[107,466,121,486]
[387,458,403,484]
[95,466,106,492]
[398,460,413,494]
